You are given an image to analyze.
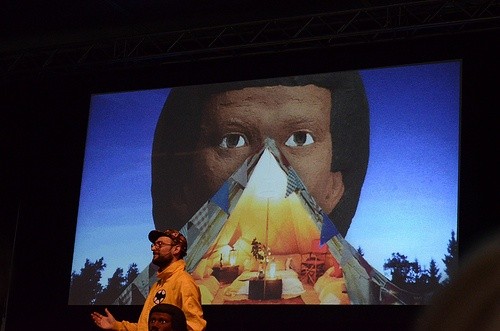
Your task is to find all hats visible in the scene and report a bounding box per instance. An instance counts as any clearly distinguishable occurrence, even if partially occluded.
[149,230,187,255]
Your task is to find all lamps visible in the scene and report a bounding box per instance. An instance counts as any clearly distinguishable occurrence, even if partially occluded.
[229,247,237,266]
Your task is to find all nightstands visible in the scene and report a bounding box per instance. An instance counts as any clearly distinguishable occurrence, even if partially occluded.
[210,265,243,284]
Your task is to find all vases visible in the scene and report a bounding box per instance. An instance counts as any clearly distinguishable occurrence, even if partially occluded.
[258,264,265,279]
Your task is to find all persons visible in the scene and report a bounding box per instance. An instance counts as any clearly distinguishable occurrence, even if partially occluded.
[147,303,187,331]
[91,229,208,331]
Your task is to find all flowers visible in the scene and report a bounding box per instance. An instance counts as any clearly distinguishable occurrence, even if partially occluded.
[250,237,271,264]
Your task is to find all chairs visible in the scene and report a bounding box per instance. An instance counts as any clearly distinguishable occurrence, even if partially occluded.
[301,239,328,286]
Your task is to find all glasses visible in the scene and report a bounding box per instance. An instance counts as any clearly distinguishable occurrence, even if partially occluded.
[151,242,173,250]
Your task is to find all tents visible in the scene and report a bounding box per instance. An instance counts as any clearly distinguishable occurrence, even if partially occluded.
[113,137,408,305]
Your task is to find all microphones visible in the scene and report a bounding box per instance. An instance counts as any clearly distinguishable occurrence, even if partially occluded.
[160,252,170,259]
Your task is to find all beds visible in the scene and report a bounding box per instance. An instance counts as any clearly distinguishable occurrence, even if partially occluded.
[223,252,306,301]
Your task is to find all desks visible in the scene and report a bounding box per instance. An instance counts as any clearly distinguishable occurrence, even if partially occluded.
[248,277,282,300]
